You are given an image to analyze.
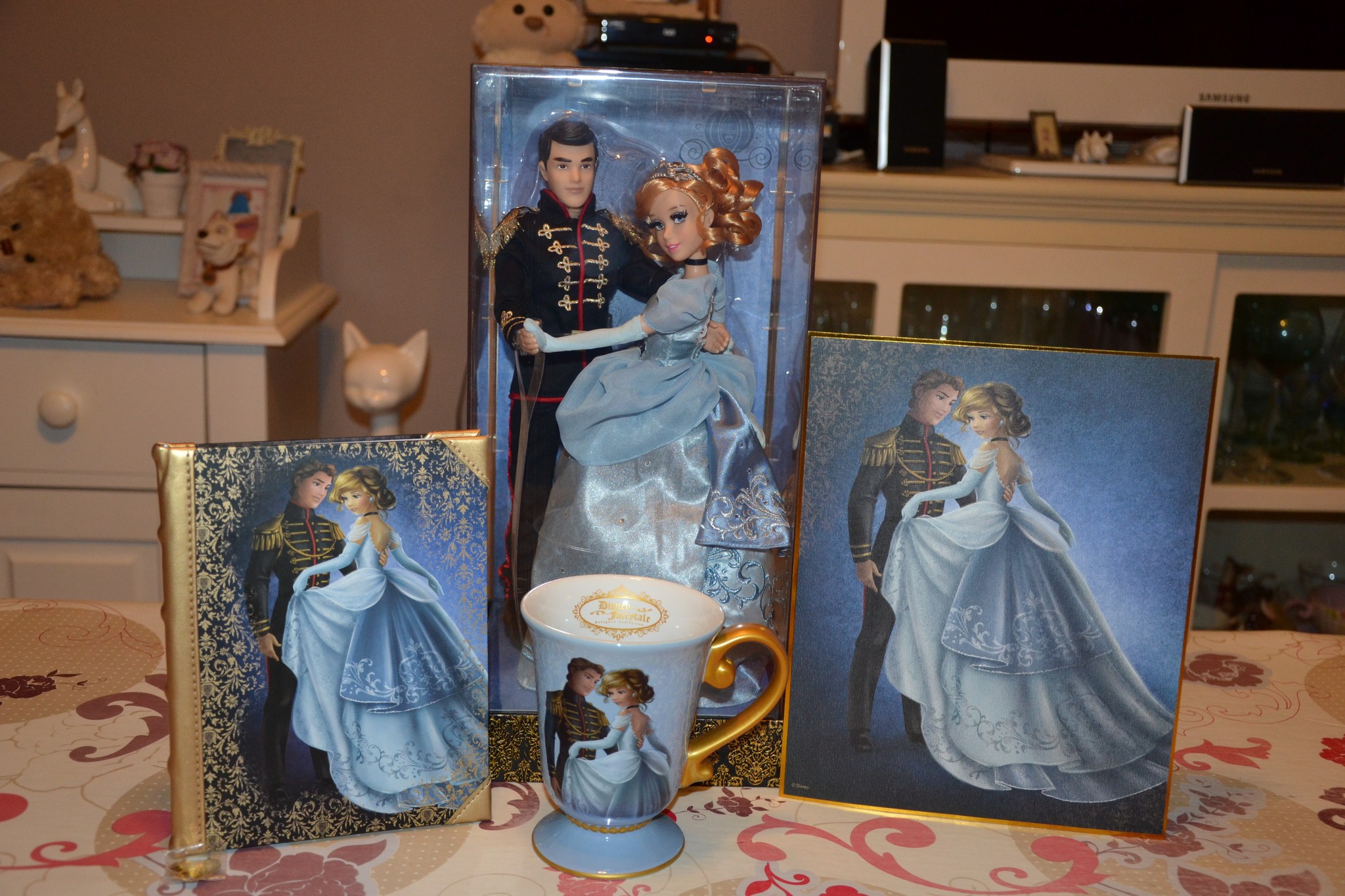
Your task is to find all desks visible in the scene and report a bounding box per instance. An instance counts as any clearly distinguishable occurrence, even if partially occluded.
[1,202,341,600]
[814,150,1345,636]
[1,599,1344,895]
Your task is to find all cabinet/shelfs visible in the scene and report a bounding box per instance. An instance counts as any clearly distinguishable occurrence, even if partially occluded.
[2,334,209,601]
[813,236,1344,635]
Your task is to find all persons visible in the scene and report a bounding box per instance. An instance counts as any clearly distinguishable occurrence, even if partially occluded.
[491,119,732,655]
[513,145,794,708]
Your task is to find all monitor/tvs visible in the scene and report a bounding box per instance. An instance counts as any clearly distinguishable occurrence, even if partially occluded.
[833,0,1345,179]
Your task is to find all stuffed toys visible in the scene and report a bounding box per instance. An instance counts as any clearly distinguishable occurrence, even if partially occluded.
[0,162,120,311]
[467,0,591,70]
[185,208,260,315]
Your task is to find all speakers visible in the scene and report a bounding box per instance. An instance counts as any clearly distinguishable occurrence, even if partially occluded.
[866,37,948,169]
[1177,102,1345,189]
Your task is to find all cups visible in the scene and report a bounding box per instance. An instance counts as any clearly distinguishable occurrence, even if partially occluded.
[513,573,791,876]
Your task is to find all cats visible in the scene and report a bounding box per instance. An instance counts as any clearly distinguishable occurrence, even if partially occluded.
[342,320,428,439]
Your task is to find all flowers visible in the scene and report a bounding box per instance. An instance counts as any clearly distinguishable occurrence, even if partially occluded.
[124,140,192,188]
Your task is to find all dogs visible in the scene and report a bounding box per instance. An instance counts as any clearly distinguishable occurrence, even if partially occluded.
[188,208,260,316]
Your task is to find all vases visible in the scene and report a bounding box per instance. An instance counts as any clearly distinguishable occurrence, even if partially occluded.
[135,169,190,221]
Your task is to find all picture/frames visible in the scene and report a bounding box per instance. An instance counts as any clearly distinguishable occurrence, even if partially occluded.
[177,126,306,310]
[777,330,1221,843]
